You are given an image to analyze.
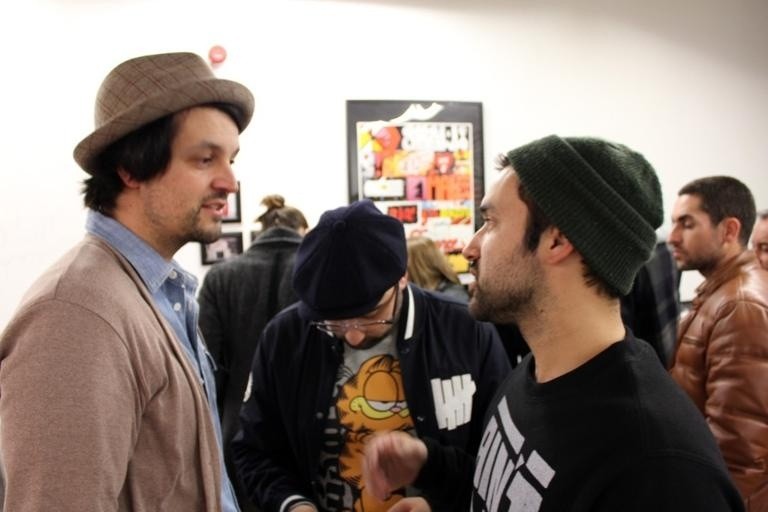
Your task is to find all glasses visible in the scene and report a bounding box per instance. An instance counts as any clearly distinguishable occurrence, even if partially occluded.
[315,282,398,332]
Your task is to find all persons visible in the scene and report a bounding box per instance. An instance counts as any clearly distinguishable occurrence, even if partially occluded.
[395,233,480,310]
[0,51,254,512]
[242,197,514,511]
[667,174,768,512]
[195,194,315,481]
[462,135,743,512]
[611,196,766,512]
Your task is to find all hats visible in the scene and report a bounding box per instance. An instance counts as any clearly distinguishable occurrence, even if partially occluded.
[507,134,663,295]
[74,53,254,175]
[292,199,408,321]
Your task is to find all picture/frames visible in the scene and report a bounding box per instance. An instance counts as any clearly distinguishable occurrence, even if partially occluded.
[199,180,244,266]
